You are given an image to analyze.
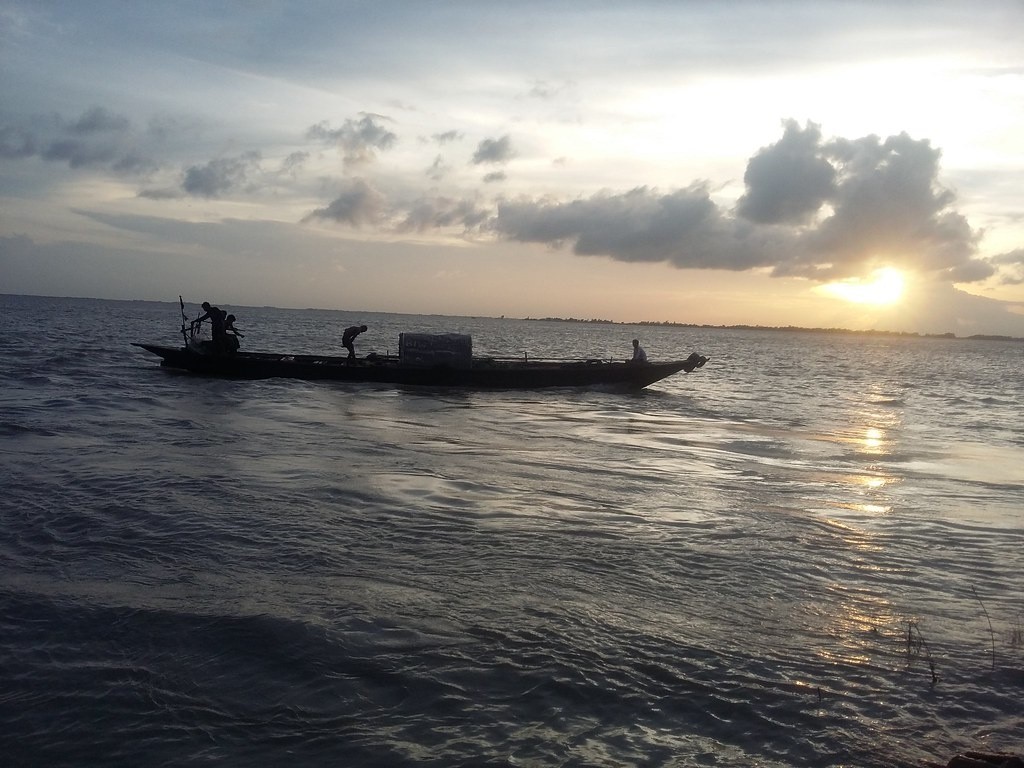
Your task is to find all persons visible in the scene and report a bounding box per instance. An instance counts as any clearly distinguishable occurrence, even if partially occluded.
[625,339,648,362]
[190,302,245,341]
[341,325,368,358]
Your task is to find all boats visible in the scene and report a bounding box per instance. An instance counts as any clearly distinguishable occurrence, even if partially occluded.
[130,296,710,390]
